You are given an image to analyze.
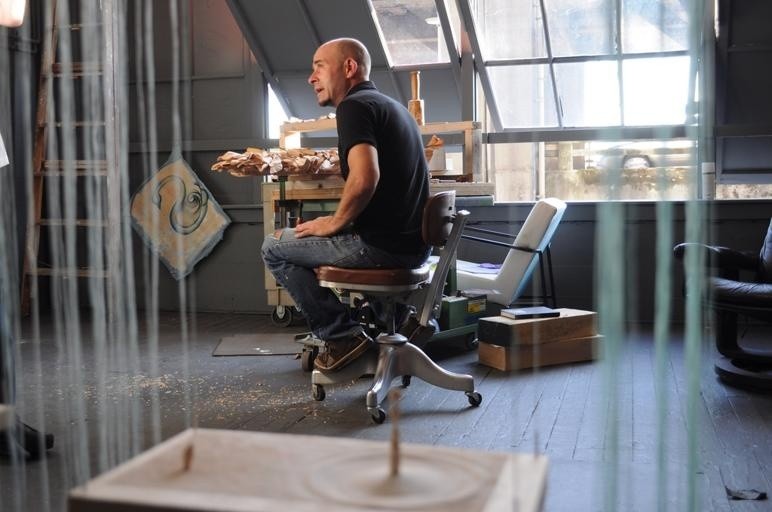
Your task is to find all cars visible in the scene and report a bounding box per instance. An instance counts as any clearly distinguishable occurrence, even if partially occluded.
[605,138,698,174]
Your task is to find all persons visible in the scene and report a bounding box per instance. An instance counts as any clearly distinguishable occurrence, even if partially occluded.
[0,0,26,28]
[259,37,432,375]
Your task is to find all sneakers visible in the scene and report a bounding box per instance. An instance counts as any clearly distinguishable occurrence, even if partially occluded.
[398,312,435,347]
[313,330,374,373]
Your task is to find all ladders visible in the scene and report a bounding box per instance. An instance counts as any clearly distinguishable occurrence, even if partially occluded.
[21,0,122,322]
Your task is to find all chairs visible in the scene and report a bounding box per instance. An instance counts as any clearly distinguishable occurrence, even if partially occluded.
[310,189,566,425]
[673,208,770,396]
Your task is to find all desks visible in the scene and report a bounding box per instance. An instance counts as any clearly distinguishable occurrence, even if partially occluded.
[261,120,496,328]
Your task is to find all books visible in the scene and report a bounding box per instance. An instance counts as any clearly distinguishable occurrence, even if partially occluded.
[501,306,559,320]
